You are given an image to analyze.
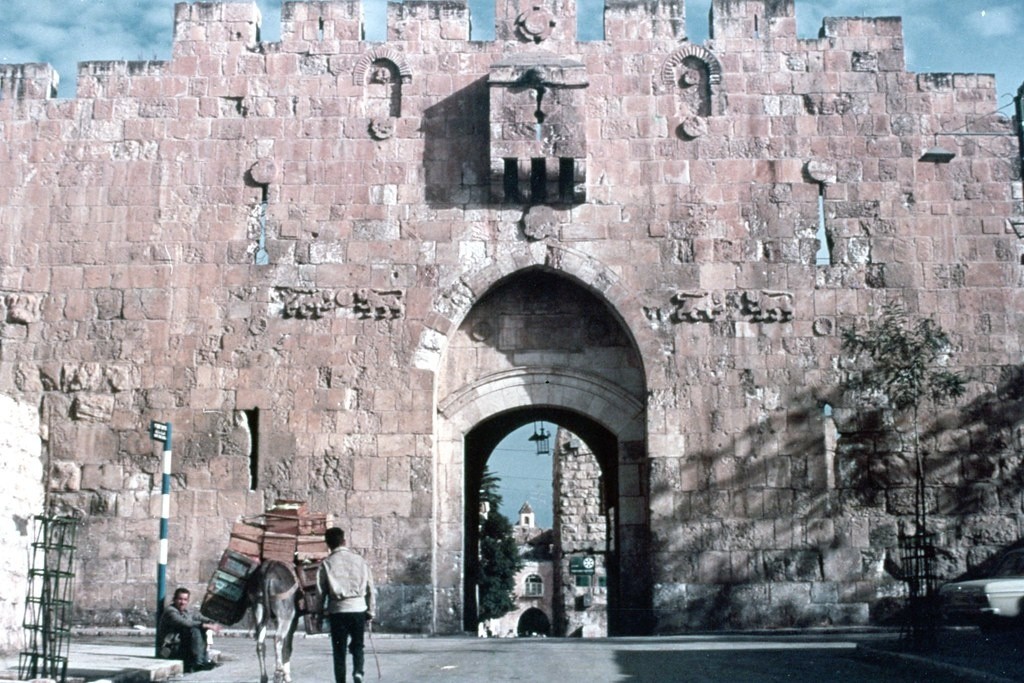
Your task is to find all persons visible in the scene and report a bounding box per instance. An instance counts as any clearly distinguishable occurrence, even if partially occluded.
[156,587,223,672]
[486,626,492,637]
[312,528,376,683]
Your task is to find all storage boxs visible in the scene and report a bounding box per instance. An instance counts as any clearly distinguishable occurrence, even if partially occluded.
[200,498,334,626]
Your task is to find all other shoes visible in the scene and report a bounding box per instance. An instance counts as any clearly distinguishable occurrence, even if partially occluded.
[193,662,215,671]
[354,672,362,683]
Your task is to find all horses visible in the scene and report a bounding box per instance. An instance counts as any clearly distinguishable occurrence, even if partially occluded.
[248,559,305,683]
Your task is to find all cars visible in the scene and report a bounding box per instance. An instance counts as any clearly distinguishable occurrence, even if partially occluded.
[939,548,1024,636]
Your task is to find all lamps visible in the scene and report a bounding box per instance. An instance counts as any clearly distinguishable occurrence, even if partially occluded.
[528,421,548,441]
